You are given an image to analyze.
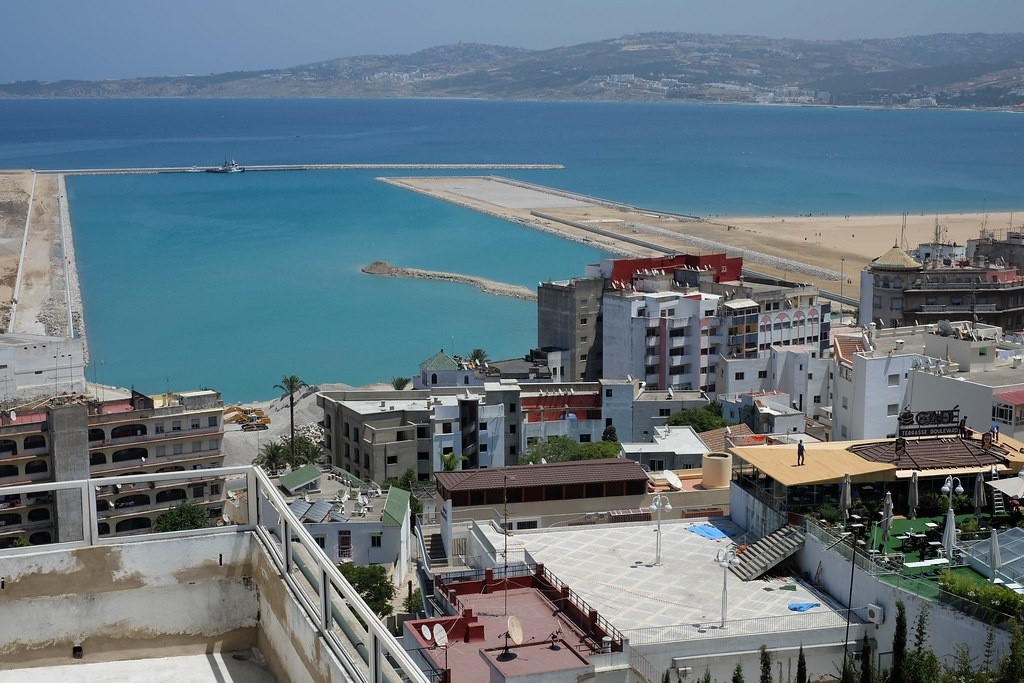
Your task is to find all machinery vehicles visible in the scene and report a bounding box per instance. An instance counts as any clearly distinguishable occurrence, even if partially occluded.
[223,406,271,432]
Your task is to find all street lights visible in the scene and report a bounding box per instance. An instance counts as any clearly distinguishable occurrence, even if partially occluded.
[941,474,964,572]
[714,547,741,629]
[649,494,673,566]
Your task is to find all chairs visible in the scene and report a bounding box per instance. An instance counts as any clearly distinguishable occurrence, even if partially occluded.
[865,527,872,537]
[871,543,884,554]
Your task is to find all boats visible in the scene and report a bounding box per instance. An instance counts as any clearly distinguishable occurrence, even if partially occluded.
[205,154,246,173]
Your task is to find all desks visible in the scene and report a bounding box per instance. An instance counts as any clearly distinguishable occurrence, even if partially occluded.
[868,550,880,554]
[903,563,921,579]
[914,534,927,549]
[928,541,941,557]
[857,540,866,548]
[924,560,940,576]
[852,523,864,535]
[987,578,1004,586]
[1013,588,1024,595]
[934,558,949,575]
[1005,583,1023,590]
[896,536,912,553]
[851,514,861,524]
[925,522,938,539]
[914,561,929,578]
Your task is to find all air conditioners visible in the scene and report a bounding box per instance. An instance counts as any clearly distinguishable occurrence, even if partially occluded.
[867,603,883,625]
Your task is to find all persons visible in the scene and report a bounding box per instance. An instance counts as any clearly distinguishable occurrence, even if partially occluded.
[798,440,806,466]
[960,416,968,440]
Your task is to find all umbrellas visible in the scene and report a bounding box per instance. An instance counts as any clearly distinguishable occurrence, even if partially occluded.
[838,474,851,529]
[973,473,987,516]
[942,508,956,571]
[990,529,1002,584]
[907,472,918,520]
[881,491,893,541]
